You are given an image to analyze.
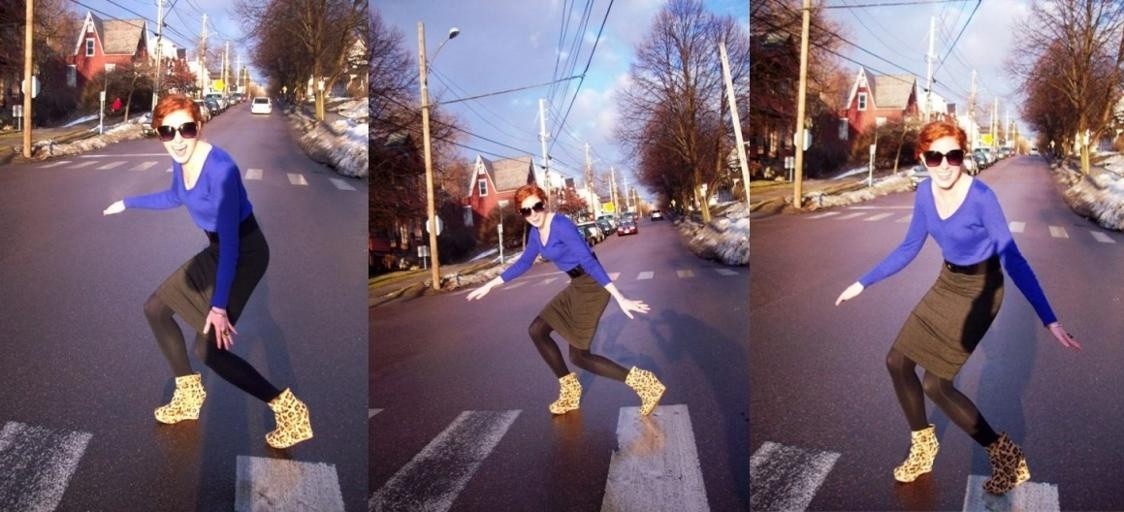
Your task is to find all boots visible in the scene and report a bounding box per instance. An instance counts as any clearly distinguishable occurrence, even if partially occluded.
[980,429,1031,496]
[892,423,942,484]
[265,387,315,451]
[623,365,668,417]
[548,370,583,415]
[152,371,209,425]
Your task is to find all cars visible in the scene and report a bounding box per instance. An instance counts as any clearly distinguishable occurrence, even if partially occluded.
[574,210,640,247]
[143,91,247,139]
[908,145,1016,196]
[252,96,273,116]
[650,210,663,220]
[1030,147,1039,156]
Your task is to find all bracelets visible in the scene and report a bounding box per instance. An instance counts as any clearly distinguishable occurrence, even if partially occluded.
[1048,322,1065,327]
[211,309,227,317]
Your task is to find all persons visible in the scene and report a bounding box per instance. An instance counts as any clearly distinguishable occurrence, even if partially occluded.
[834,123,1081,495]
[465,185,667,416]
[101,94,313,449]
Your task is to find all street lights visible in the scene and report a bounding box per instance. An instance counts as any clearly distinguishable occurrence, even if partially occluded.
[415,19,460,293]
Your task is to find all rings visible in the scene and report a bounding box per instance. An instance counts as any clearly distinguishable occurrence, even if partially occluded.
[1066,333,1074,338]
[223,328,229,338]
[639,307,642,309]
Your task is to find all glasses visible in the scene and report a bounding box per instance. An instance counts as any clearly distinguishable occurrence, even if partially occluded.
[519,202,546,217]
[155,121,199,141]
[922,149,967,167]
